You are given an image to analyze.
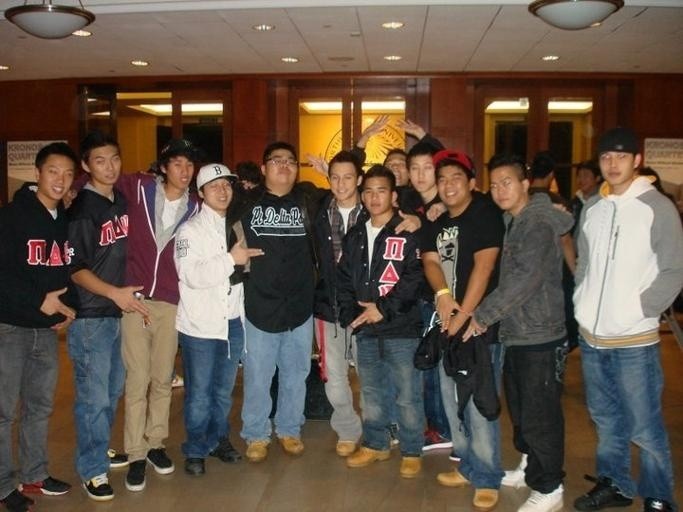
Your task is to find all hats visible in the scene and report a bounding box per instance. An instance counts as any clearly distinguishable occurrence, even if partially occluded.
[158,140,206,164]
[432,148,477,178]
[599,129,638,154]
[197,163,239,192]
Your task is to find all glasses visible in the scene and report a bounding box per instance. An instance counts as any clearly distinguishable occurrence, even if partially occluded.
[264,158,298,167]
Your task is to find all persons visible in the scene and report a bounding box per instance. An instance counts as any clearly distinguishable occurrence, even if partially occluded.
[60,123,151,502]
[637,164,681,322]
[63,135,204,492]
[570,127,683,510]
[145,114,603,510]
[0,141,80,510]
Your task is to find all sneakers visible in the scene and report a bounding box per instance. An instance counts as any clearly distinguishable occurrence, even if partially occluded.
[147,447,175,475]
[277,436,304,458]
[125,458,147,492]
[472,488,499,512]
[574,484,632,512]
[184,458,205,478]
[18,476,71,496]
[209,439,243,464]
[436,470,471,487]
[108,448,130,468]
[500,468,529,488]
[335,424,461,479]
[245,441,269,463]
[0,489,36,511]
[82,473,114,502]
[518,484,563,512]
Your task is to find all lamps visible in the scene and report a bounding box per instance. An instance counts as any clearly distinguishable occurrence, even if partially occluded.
[4,0,96,39]
[529,0,624,31]
